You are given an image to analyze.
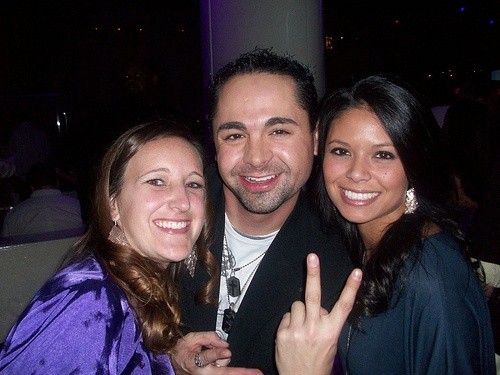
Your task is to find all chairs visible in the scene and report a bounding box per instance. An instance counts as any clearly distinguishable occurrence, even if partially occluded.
[0,225,85,345]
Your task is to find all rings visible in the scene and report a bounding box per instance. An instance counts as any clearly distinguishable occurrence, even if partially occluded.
[193,352,211,368]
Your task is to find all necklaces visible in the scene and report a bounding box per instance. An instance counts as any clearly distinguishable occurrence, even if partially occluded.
[343,248,366,373]
[220,229,267,336]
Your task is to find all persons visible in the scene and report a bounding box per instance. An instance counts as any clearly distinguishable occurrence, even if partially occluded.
[160,47,366,375]
[308,74,497,375]
[0,108,86,237]
[0,118,220,375]
